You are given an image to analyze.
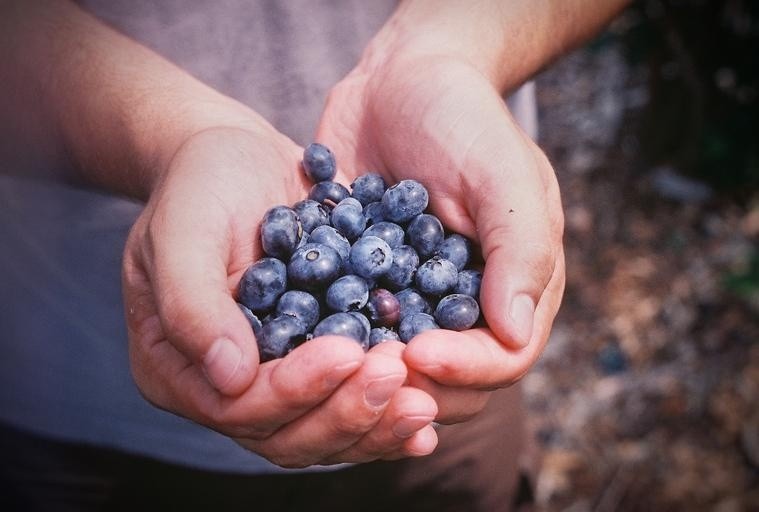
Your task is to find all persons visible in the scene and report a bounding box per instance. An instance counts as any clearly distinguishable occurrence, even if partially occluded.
[0,0,628,512]
